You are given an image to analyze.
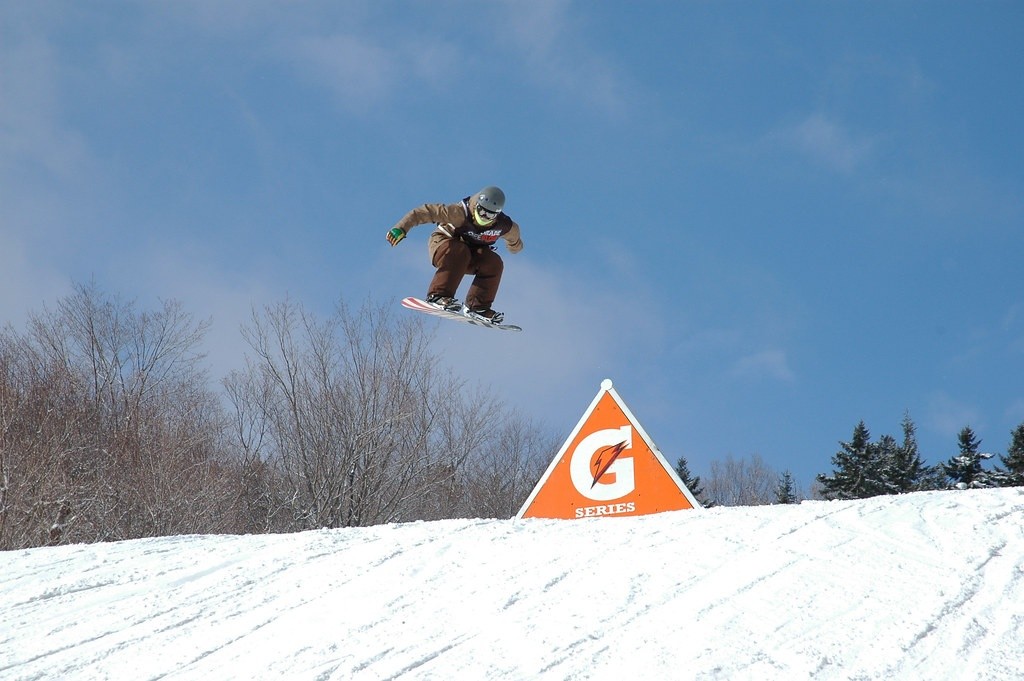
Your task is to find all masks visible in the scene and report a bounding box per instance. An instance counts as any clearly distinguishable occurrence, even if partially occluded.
[474,209,494,226]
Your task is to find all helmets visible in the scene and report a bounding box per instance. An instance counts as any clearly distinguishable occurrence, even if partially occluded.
[473,186,505,217]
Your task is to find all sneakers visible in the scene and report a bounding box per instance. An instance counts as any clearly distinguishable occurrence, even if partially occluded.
[425,295,464,314]
[463,304,504,321]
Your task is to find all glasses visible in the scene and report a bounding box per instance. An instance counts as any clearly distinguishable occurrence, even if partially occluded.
[477,202,499,220]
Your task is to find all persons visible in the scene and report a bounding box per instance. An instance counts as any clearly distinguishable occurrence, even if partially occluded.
[386,186,522,322]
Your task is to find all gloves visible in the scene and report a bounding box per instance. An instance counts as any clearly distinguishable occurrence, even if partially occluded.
[386,227,406,246]
[508,241,523,254]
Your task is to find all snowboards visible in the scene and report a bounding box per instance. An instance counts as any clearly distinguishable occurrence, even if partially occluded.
[402,296,522,332]
